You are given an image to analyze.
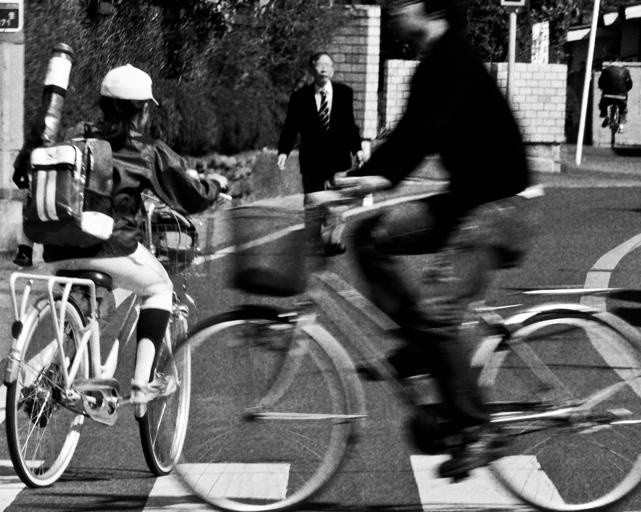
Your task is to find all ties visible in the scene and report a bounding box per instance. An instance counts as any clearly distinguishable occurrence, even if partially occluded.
[318,90,331,129]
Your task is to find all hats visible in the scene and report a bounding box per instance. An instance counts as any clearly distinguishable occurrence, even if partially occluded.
[100,63,161,108]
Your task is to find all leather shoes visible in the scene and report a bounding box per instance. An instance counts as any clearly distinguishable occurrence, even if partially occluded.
[355,340,430,383]
[434,425,515,480]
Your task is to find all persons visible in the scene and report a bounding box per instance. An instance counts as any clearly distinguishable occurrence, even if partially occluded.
[277,50,366,240]
[23,54,231,433]
[334,0,541,478]
[9,133,44,264]
[595,60,634,133]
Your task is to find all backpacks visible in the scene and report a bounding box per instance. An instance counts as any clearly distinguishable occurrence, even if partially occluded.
[9,120,117,249]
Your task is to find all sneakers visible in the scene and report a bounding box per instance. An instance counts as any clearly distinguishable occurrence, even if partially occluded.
[601,116,625,134]
[129,370,179,407]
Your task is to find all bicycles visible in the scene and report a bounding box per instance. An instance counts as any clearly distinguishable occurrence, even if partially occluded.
[150,168,640,512]
[606,102,624,147]
[3,184,233,487]
